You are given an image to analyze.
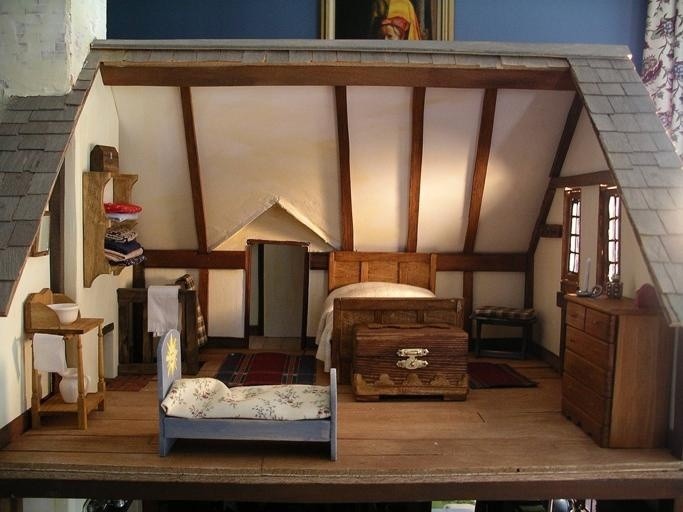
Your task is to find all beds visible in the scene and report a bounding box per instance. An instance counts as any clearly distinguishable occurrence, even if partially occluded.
[155,329,341,463]
[324,249,464,386]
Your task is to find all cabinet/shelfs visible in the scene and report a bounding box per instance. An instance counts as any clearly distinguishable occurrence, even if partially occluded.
[81,171,139,287]
[22,287,106,431]
[561,292,667,449]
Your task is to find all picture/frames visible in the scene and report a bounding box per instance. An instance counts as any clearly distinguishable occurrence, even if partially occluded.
[318,1,455,43]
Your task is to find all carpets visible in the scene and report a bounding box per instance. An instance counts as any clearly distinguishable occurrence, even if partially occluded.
[208,349,318,386]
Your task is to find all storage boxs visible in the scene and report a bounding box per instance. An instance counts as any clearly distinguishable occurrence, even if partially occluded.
[349,322,469,402]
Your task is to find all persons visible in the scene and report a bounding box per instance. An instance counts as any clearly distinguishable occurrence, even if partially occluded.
[368,1,421,41]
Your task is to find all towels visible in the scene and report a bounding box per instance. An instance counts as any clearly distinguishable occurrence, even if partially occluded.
[147,283,182,338]
[29,333,67,374]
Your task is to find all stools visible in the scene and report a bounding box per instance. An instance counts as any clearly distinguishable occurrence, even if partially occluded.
[469,303,538,360]
